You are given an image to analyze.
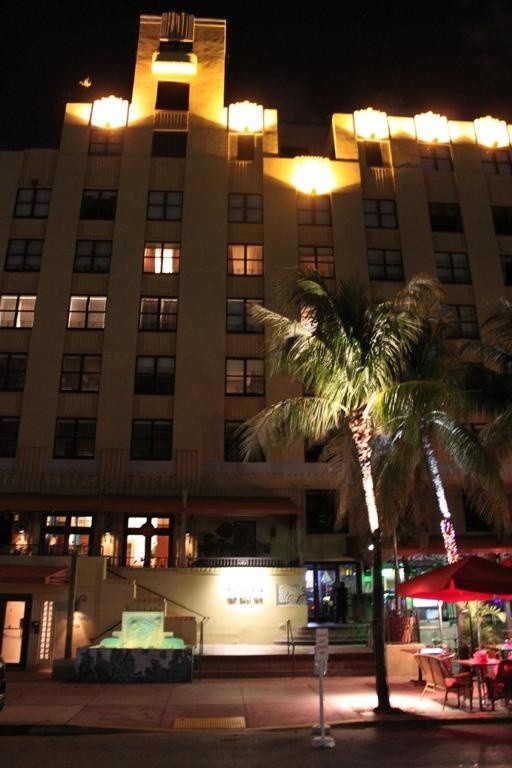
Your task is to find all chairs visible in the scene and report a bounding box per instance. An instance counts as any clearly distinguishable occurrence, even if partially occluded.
[412,652,512,713]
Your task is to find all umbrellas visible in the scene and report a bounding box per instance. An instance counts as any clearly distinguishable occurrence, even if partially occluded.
[394,554,512,656]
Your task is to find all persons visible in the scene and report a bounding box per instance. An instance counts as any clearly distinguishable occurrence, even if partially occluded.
[334,581,350,624]
